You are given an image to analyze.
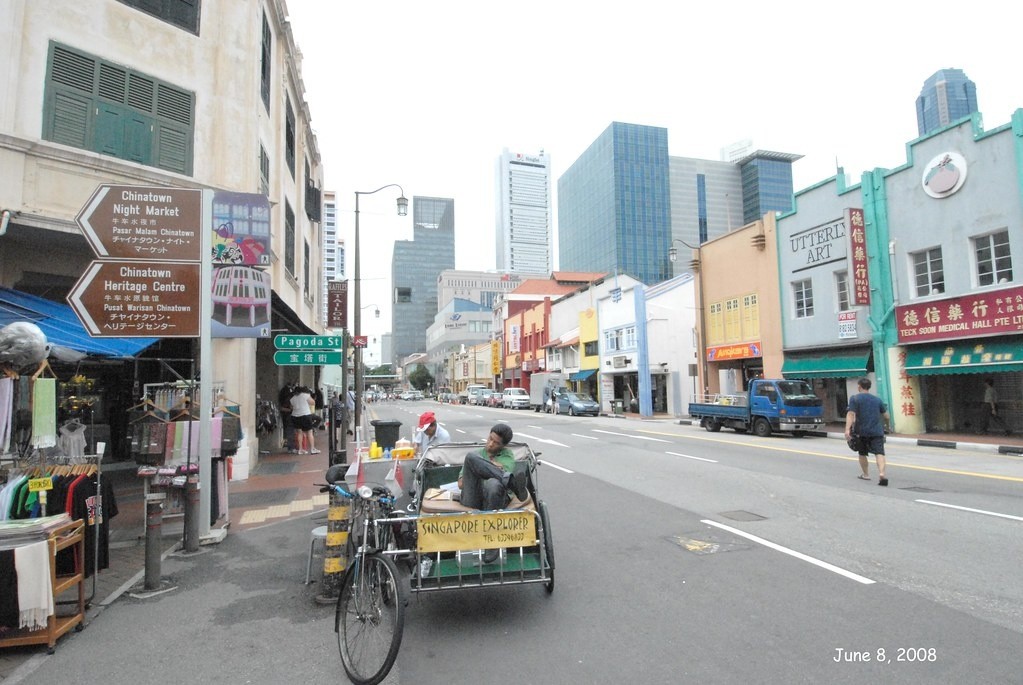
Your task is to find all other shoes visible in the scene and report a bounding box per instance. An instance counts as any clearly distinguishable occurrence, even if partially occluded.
[298,450,308,454]
[288,448,298,454]
[347,429,353,435]
[513,472,528,501]
[484,549,499,562]
[311,449,320,453]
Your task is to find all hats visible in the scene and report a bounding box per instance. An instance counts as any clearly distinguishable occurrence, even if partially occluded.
[416,412,435,431]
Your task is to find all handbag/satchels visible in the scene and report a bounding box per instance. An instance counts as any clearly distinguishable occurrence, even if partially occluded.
[312,414,322,428]
[847,431,861,450]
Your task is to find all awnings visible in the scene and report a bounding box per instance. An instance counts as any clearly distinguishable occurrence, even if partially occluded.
[906,332,1023,375]
[570,369,597,380]
[780,345,871,378]
[0,284,161,360]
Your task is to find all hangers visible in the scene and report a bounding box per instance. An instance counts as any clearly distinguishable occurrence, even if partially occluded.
[0,353,63,382]
[59,418,87,435]
[126,379,245,428]
[0,455,112,495]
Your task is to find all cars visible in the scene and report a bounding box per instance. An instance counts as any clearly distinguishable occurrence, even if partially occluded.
[393,372,600,417]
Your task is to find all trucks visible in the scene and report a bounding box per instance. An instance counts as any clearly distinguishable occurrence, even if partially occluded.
[688,377,824,438]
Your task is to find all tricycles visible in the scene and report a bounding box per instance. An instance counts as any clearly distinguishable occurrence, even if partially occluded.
[312,440,557,685]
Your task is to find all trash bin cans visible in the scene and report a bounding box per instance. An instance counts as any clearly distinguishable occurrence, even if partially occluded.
[610,399,623,414]
[370,419,403,452]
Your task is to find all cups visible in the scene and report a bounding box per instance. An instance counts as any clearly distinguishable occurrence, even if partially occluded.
[370,442,382,459]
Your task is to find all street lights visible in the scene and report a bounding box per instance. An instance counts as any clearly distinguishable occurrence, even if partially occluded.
[353,183,409,434]
[669,239,710,405]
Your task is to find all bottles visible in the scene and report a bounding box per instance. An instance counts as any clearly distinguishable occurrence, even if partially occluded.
[389,447,395,458]
[358,485,372,498]
[383,447,389,458]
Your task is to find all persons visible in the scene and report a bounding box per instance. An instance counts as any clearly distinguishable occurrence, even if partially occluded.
[279,384,322,454]
[411,410,450,457]
[551,388,556,414]
[329,390,339,414]
[380,394,388,401]
[347,386,355,436]
[976,377,998,436]
[335,393,344,443]
[844,379,892,486]
[458,423,529,563]
[361,394,366,414]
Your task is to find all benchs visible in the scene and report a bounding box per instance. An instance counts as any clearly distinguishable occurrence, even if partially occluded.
[417,460,538,519]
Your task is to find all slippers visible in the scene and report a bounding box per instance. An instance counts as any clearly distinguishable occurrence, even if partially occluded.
[858,474,870,480]
[879,479,888,486]
